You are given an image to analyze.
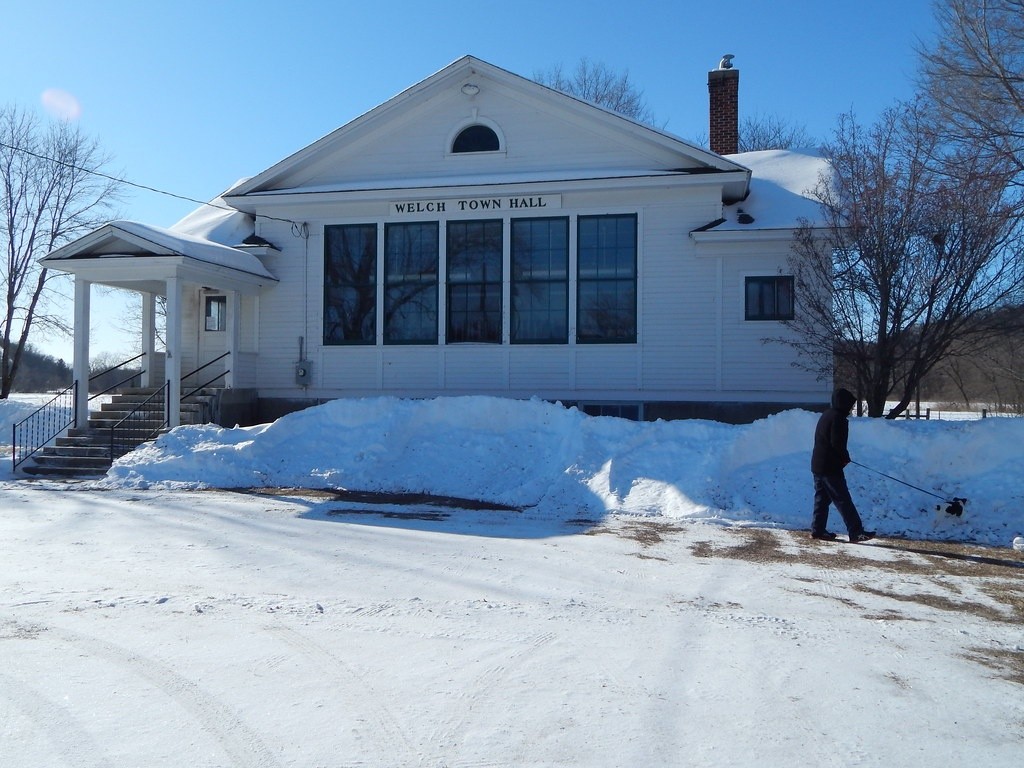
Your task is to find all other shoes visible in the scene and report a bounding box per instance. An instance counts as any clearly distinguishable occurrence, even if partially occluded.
[849,528,877,543]
[813,529,836,540]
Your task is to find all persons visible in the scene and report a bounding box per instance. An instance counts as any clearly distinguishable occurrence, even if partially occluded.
[812,388,876,543]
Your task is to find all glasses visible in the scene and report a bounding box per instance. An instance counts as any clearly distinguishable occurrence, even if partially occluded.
[852,404,855,407]
